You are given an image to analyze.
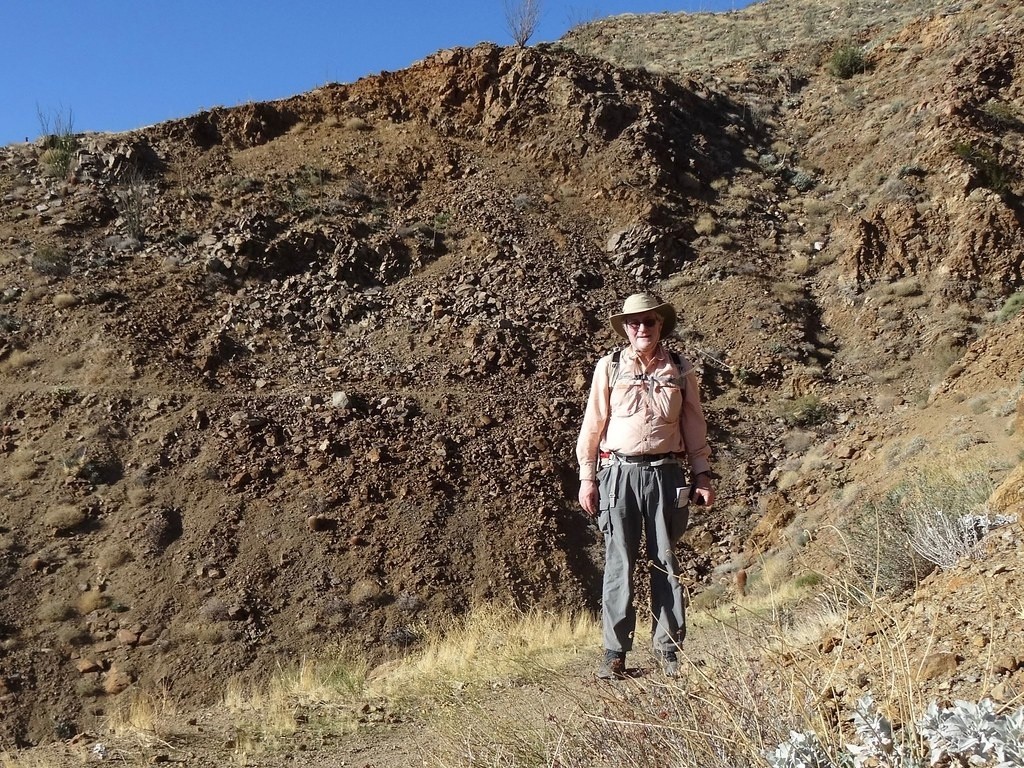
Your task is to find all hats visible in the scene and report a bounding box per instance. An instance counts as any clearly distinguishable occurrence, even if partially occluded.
[610,293,676,341]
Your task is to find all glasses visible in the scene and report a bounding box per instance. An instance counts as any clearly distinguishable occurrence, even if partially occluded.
[627,318,658,330]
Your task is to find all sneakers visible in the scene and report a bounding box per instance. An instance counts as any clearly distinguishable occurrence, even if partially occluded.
[597,650,626,679]
[654,650,684,678]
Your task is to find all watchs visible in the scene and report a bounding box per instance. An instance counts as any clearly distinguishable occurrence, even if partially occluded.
[703,470,712,478]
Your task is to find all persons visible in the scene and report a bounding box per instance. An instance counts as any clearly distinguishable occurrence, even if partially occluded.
[575,292,717,680]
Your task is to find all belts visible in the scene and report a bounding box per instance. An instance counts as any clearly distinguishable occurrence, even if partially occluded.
[601,450,688,463]
